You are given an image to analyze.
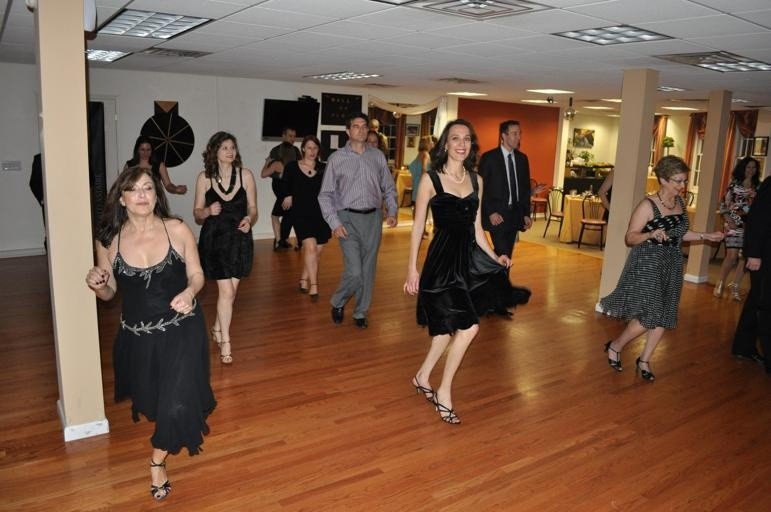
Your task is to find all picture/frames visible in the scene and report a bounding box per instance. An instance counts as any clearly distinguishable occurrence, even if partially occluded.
[405,123,420,137]
[752,136,768,157]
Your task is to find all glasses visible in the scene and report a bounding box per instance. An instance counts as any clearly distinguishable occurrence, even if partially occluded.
[662,175,690,184]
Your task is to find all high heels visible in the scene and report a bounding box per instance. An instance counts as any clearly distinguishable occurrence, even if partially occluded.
[604,340,625,371]
[274,238,287,250]
[210,327,222,347]
[714,279,725,298]
[636,356,657,381]
[150,458,172,501]
[298,277,310,294]
[433,391,461,425]
[412,373,435,403]
[727,280,744,302]
[309,282,319,302]
[220,340,233,363]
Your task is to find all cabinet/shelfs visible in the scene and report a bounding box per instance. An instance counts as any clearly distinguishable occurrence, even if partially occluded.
[561,164,615,221]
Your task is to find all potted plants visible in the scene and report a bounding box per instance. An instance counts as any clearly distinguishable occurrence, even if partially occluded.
[577,150,593,166]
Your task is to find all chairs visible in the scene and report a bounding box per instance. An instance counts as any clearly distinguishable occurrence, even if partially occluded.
[577,192,608,251]
[542,188,565,238]
[529,178,547,223]
[681,190,694,206]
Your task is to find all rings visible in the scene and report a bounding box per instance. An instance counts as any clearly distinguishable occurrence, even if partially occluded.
[659,233,663,238]
[656,234,658,240]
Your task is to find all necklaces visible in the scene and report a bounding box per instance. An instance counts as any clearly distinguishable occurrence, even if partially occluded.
[656,191,676,209]
[215,166,236,195]
[302,163,315,175]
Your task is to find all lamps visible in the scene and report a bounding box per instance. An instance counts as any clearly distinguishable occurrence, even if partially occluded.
[547,97,553,104]
[562,96,580,121]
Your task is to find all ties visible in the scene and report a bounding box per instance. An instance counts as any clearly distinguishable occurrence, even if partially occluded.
[508,153,518,212]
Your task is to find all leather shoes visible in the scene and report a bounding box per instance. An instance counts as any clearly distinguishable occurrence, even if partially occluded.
[331,304,344,324]
[274,238,293,249]
[731,347,771,368]
[355,317,369,329]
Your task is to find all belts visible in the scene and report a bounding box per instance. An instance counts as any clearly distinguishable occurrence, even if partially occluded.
[343,207,378,214]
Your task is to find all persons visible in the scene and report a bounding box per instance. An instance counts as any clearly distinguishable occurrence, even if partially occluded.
[86,167,219,500]
[597,169,614,224]
[261,141,296,249]
[600,155,724,381]
[261,128,302,249]
[403,119,515,426]
[404,122,533,319]
[123,136,187,194]
[318,116,398,328]
[278,135,332,301]
[193,131,258,364]
[30,153,47,253]
[714,157,762,302]
[731,175,771,374]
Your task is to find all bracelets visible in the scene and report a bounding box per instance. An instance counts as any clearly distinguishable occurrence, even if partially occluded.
[700,232,704,240]
[244,216,251,222]
